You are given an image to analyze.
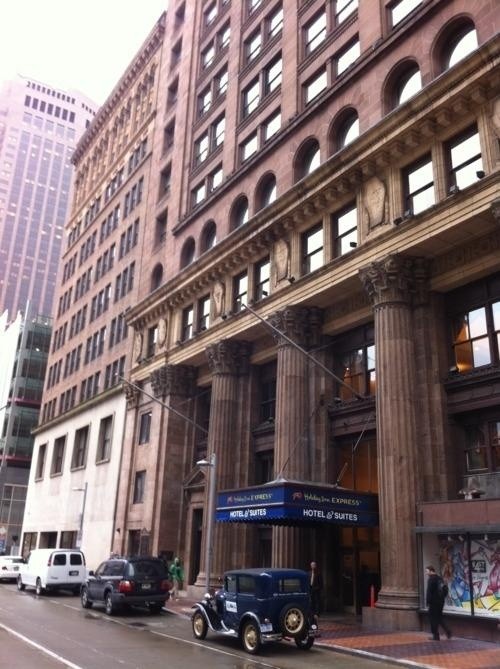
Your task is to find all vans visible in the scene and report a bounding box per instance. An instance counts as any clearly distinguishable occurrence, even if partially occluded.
[15,548,78,596]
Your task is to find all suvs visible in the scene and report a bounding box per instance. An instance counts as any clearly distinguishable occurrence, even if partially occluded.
[80,556,173,617]
[190,567,321,655]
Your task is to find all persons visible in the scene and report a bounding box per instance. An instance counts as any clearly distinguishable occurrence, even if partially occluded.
[168,556,185,602]
[425,565,452,641]
[307,561,322,618]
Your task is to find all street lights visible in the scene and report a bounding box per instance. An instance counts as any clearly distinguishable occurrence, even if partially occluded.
[72,480,88,550]
[195,452,217,593]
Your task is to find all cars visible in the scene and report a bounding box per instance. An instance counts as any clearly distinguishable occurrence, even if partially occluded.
[1,555,27,579]
